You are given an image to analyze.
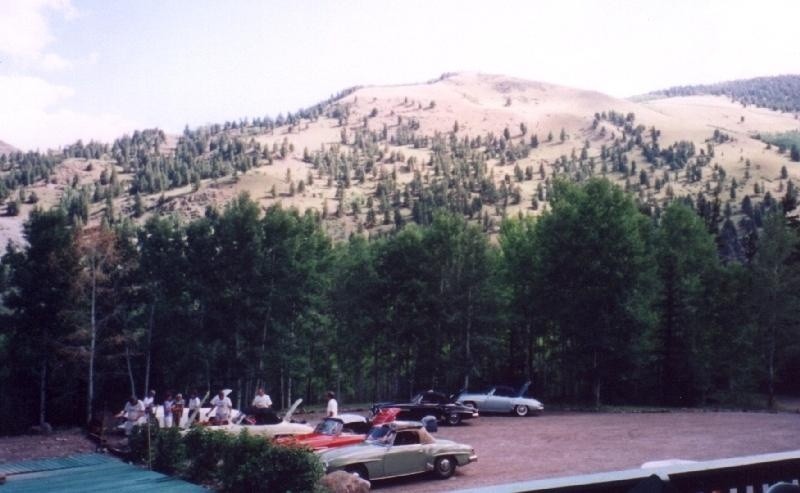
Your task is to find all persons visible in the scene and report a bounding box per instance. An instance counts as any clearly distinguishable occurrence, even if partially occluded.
[162,393,176,430]
[186,389,203,422]
[171,392,186,428]
[143,388,159,429]
[250,387,275,424]
[204,391,234,420]
[119,395,145,436]
[323,389,340,416]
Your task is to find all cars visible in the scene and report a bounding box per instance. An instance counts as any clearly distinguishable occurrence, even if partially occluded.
[116,388,247,430]
[314,420,480,488]
[172,397,313,445]
[365,388,480,427]
[268,406,402,455]
[447,381,543,418]
